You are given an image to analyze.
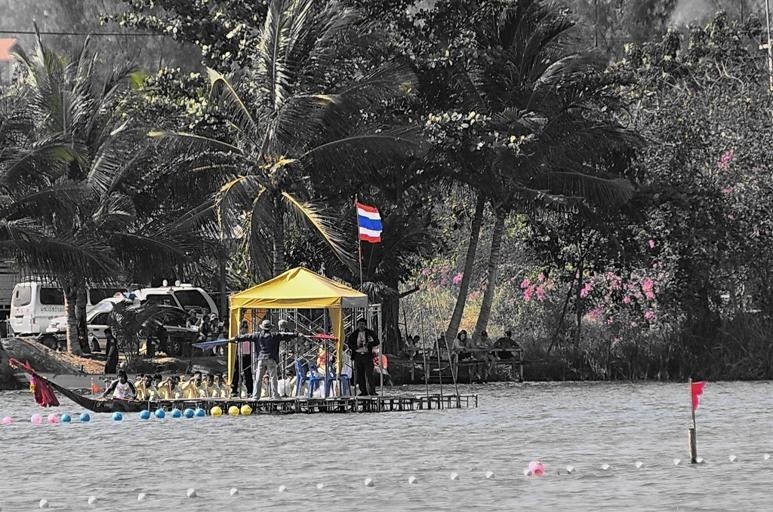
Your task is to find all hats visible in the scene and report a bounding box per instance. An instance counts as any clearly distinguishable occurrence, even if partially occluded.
[259,320,272,331]
[210,313,218,321]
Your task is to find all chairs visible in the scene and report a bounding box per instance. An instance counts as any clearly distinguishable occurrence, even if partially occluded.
[295,359,352,398]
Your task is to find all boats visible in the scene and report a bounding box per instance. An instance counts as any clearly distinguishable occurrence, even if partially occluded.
[7,357,479,410]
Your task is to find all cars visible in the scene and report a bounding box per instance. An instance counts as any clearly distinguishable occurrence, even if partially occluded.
[47,283,221,350]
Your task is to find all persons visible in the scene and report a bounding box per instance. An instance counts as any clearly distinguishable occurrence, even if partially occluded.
[134,369,340,404]
[372,345,394,390]
[97,370,137,402]
[347,319,380,396]
[230,319,255,397]
[403,328,521,379]
[196,310,230,358]
[329,343,352,381]
[104,327,118,373]
[228,319,303,400]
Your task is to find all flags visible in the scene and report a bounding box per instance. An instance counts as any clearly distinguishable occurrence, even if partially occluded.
[355,193,384,242]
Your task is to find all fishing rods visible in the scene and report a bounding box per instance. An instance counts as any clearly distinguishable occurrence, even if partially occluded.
[434,288,460,409]
[420,297,428,400]
[431,293,443,410]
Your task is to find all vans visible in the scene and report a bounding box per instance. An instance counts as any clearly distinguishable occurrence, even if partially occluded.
[10,274,152,337]
